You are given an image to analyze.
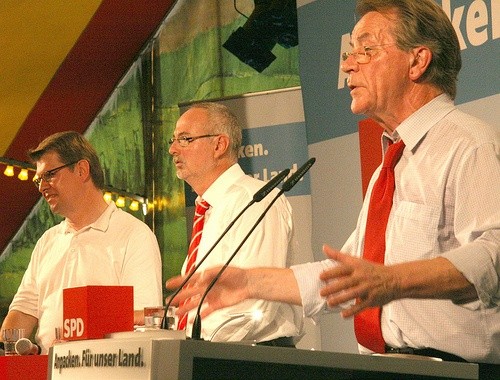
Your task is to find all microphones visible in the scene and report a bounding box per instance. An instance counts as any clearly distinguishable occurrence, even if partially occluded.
[160,157,317,339]
[14,338,42,356]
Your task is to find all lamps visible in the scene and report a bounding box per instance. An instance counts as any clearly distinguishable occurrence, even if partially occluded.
[127,194,141,211]
[103,185,113,203]
[3,158,16,177]
[17,160,30,181]
[115,189,127,208]
[222,0,298,74]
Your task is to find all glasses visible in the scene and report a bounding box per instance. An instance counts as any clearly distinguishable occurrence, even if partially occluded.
[169,134,219,147]
[342,41,399,65]
[33,158,84,189]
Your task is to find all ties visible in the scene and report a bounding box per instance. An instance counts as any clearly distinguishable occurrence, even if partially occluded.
[176,200,210,331]
[354,139,406,354]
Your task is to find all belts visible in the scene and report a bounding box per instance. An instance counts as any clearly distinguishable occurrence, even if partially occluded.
[257,337,295,347]
[385,347,466,362]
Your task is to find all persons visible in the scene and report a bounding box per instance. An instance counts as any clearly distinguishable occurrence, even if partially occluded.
[165,0,500,380]
[169,102,304,348]
[0,131,163,356]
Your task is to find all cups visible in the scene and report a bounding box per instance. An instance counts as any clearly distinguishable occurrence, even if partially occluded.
[143,306,175,331]
[3,328,26,357]
